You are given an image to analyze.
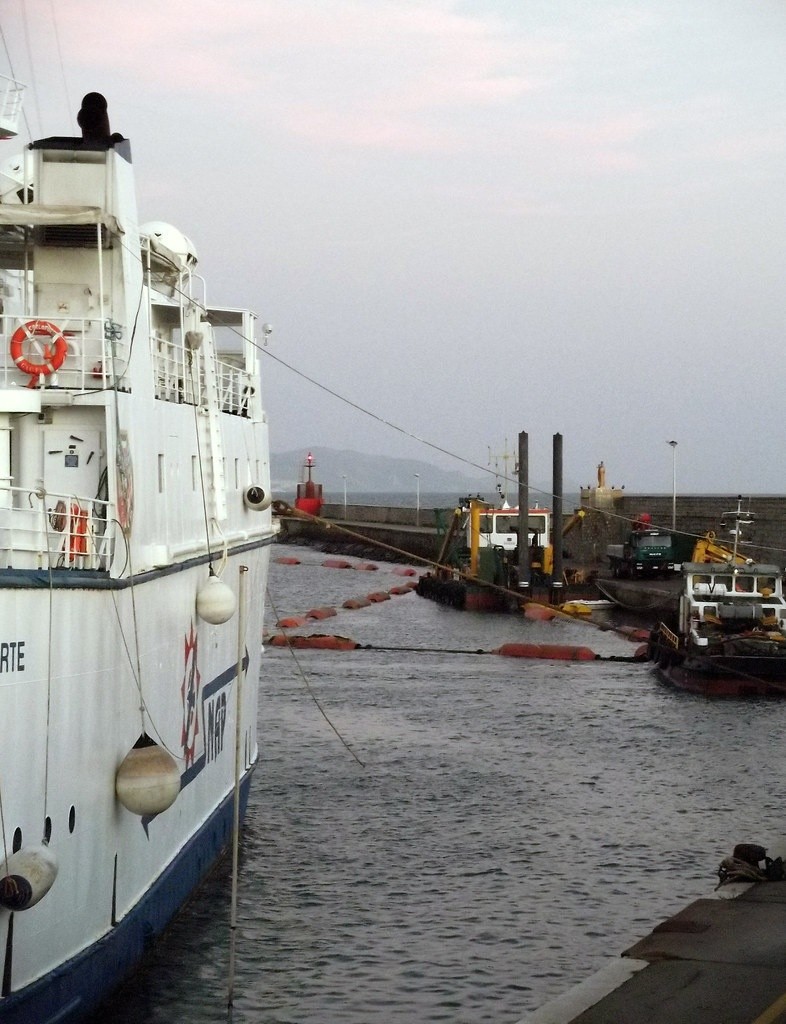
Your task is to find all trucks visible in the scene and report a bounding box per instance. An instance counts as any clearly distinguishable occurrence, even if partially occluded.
[606,526,689,581]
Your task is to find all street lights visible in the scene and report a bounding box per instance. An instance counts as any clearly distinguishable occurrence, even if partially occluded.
[342,474,347,520]
[414,473,421,527]
[669,439,677,531]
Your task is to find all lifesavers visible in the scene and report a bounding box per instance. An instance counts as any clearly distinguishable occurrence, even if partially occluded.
[10,320,67,376]
[438,563,452,584]
[61,503,81,561]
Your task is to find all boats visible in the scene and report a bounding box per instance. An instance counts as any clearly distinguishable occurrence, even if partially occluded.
[648,495,786,696]
[293,451,326,518]
[0,93,276,1024]
[421,436,587,611]
